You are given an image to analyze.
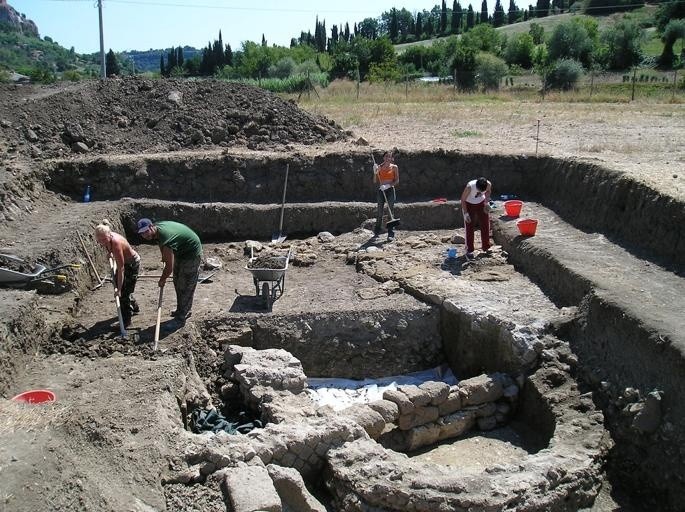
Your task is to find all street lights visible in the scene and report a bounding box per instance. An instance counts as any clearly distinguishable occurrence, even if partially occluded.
[344,52,359,69]
[128,54,135,74]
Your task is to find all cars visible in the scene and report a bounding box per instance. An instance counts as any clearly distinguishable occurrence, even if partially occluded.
[0,63,30,83]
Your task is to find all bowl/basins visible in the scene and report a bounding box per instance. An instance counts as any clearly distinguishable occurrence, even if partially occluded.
[10,389,57,405]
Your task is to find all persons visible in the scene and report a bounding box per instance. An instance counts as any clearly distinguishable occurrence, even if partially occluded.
[135,217,203,333]
[371,150,400,243]
[460,177,494,261]
[93,218,141,330]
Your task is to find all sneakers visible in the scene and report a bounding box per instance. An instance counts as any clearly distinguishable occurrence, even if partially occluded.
[485,248,493,254]
[467,252,474,257]
[166,311,191,330]
[387,237,393,241]
[116,323,133,329]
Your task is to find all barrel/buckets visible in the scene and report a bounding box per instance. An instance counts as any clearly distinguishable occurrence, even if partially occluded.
[8,389,58,404]
[516,217,538,237]
[504,199,523,218]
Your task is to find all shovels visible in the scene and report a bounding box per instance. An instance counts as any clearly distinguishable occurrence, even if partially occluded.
[109,256,133,343]
[137,271,217,284]
[271,162,289,246]
[147,262,169,357]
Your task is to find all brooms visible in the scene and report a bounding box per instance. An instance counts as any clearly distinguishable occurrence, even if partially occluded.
[370,153,401,228]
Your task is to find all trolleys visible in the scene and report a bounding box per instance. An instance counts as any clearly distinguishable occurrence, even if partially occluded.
[0,251,82,291]
[242,241,292,316]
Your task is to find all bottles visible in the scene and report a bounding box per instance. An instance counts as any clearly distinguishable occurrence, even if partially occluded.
[500,194,518,201]
[83,184,91,202]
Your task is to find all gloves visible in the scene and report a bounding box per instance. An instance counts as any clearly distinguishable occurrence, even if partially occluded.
[373,164,379,174]
[464,213,471,223]
[379,184,391,191]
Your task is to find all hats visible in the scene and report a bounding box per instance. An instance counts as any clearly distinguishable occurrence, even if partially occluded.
[138,219,152,233]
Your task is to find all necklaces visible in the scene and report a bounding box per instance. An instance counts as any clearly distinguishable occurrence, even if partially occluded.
[105,235,112,254]
